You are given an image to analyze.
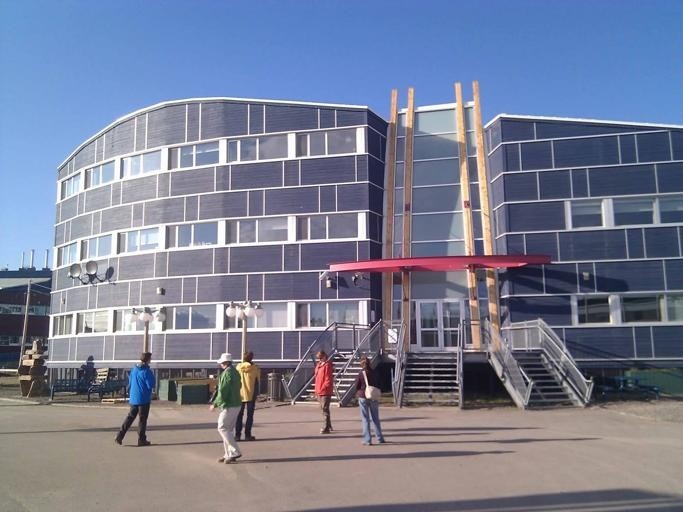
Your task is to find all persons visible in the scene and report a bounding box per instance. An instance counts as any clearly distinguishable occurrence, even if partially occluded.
[113,351,156,445]
[353,355,384,446]
[209,352,243,465]
[234,349,261,441]
[313,349,334,434]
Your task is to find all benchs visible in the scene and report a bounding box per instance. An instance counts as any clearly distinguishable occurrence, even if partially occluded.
[49,379,128,402]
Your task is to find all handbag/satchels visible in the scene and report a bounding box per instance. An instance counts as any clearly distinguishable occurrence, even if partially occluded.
[364,385,381,401]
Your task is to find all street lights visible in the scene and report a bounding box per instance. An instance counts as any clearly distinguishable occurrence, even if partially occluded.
[129,305,170,355]
[223,299,264,364]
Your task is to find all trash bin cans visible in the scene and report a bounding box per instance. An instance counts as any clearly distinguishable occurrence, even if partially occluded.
[267,373,282,401]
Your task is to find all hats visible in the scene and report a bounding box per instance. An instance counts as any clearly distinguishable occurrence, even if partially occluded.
[216,353,234,364]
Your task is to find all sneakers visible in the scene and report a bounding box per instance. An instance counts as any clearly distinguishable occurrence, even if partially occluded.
[377,438,384,443]
[319,428,329,433]
[244,435,254,440]
[137,439,150,445]
[234,436,239,440]
[223,454,241,464]
[361,440,370,445]
[114,434,121,445]
[218,455,235,461]
